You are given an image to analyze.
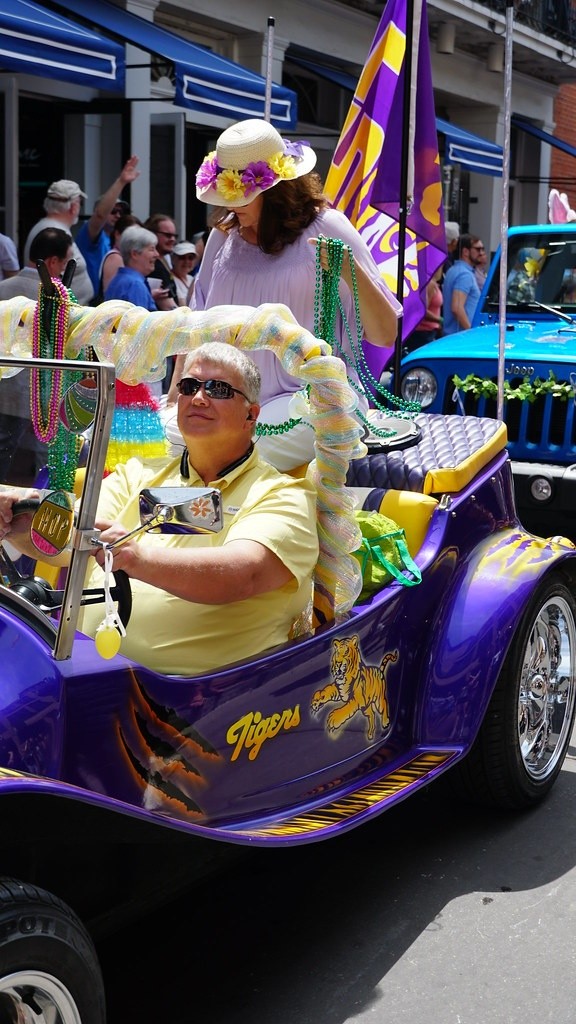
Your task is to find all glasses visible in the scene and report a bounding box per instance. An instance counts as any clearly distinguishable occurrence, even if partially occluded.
[471,247,484,252]
[178,255,195,260]
[111,207,125,215]
[157,231,179,240]
[176,377,252,404]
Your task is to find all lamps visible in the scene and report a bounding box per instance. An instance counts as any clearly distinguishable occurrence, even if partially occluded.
[435,22,456,56]
[486,45,505,72]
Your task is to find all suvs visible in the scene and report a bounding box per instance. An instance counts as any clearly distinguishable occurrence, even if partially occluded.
[369,223,575,543]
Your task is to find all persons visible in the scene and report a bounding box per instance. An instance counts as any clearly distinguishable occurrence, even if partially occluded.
[507,247,541,299]
[0,232,19,281]
[0,228,75,486]
[0,339,319,672]
[407,222,488,351]
[153,504,159,522]
[139,497,151,526]
[209,492,220,526]
[26,156,211,395]
[160,119,402,468]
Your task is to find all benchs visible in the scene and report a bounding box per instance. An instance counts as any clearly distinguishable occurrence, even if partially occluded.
[21,468,446,637]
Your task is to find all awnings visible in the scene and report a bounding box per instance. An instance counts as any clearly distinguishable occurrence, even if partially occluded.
[0,0,123,91]
[288,53,504,177]
[511,119,576,191]
[56,0,297,130]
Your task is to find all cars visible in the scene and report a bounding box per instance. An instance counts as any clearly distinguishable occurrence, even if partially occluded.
[0,358,575,1024]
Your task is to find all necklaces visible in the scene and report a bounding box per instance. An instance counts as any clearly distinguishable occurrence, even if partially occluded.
[254,234,421,438]
[28,279,95,492]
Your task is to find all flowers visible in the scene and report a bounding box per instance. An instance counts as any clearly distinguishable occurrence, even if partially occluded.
[195,139,312,199]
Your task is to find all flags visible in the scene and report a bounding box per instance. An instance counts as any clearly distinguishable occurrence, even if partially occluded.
[325,0,449,398]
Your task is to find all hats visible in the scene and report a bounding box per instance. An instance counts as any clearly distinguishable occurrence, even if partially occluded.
[47,179,88,202]
[95,194,129,206]
[172,242,198,256]
[195,118,317,207]
